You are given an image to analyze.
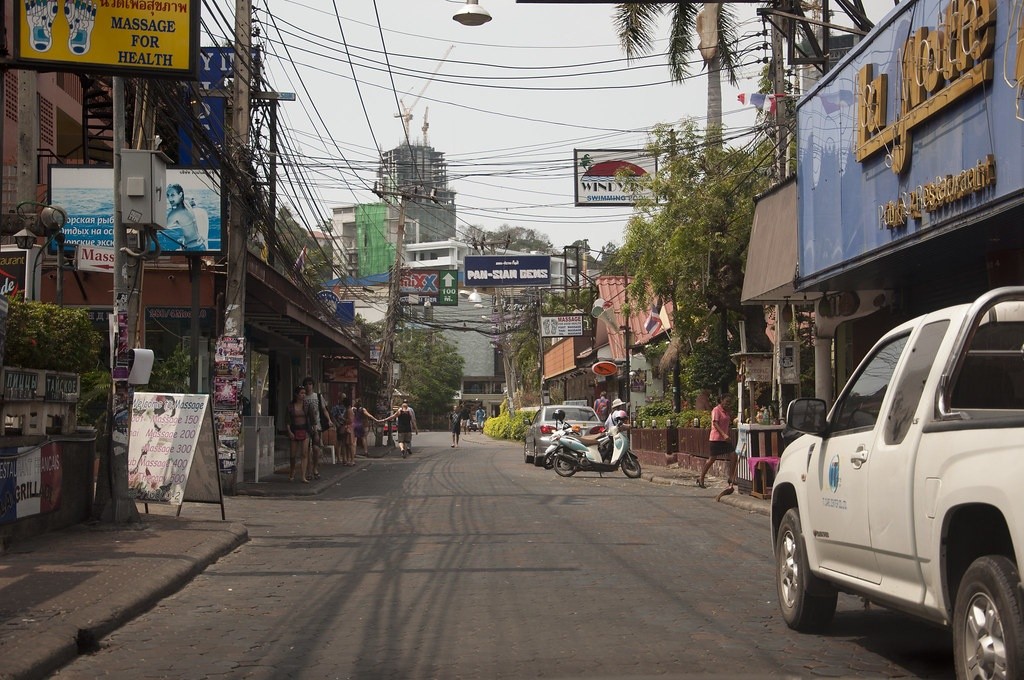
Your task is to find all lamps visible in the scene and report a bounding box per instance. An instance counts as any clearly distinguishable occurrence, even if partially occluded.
[693,418,700,427]
[633,420,637,428]
[666,419,671,429]
[468,290,482,304]
[652,420,656,428]
[445,0,492,26]
[642,420,646,428]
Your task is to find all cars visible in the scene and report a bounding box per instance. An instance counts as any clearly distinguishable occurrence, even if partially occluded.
[469,421,484,431]
[384,423,397,435]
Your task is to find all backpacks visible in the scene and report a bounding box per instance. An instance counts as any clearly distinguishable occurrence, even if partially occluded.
[596,398,609,421]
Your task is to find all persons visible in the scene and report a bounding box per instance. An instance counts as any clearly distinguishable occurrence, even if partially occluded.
[446,403,487,449]
[696,392,739,490]
[166,183,207,251]
[329,391,418,467]
[593,391,633,434]
[285,377,334,485]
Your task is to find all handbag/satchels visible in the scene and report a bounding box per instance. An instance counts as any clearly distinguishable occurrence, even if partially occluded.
[294,429,307,441]
[317,392,330,432]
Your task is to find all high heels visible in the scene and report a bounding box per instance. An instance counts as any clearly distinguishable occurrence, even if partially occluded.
[727,478,738,486]
[695,478,706,489]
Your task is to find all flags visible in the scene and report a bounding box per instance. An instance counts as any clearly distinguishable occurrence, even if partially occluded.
[292,245,307,275]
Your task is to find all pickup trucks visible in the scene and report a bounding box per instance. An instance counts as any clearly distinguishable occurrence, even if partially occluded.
[770,284,1024,680]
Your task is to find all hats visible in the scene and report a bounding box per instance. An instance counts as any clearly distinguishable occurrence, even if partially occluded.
[340,392,346,398]
[611,398,626,409]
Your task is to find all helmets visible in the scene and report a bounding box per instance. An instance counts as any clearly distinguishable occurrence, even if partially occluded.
[552,410,566,421]
[613,409,628,420]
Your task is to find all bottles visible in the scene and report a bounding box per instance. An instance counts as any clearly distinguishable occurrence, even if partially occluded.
[763,409,770,425]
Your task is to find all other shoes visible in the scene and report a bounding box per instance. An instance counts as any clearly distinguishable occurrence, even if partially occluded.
[400,449,408,459]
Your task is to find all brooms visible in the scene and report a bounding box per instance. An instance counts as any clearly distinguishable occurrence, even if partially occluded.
[715,442,746,502]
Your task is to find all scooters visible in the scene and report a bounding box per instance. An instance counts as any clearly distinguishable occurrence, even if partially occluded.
[524,403,604,466]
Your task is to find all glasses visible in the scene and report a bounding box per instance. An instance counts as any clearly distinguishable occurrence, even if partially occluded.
[304,383,312,385]
[295,386,305,392]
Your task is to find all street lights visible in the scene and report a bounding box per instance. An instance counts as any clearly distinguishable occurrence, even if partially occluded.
[386,296,431,448]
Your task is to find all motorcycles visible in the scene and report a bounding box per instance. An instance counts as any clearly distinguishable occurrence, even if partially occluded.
[543,409,642,479]
